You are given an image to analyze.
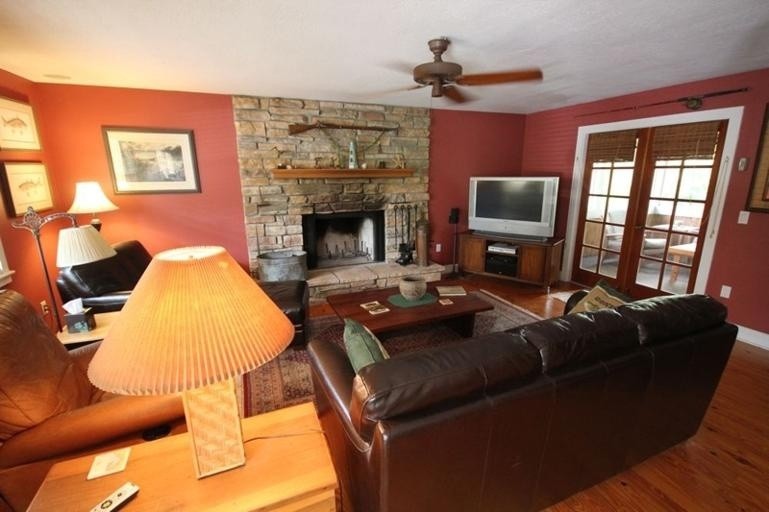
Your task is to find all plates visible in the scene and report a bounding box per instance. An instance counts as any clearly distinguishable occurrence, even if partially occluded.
[387,293,438,308]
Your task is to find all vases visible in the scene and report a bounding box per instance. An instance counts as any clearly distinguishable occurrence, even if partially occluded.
[399,277,427,300]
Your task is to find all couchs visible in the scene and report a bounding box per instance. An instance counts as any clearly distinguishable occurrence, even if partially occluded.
[564,291,738,512]
[0,289,186,512]
[308,331,557,512]
[597,211,668,273]
[513,321,655,512]
[56,241,310,350]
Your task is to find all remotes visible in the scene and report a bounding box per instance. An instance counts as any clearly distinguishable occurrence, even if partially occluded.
[87,480,140,512]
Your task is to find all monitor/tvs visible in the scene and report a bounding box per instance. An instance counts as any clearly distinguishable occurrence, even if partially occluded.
[468,176,560,242]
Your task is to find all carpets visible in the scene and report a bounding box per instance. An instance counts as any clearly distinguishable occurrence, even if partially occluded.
[244,289,544,417]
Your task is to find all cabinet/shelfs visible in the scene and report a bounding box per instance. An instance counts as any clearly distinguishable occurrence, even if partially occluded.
[459,231,565,294]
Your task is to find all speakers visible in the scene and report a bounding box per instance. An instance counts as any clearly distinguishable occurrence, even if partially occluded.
[485,255,518,276]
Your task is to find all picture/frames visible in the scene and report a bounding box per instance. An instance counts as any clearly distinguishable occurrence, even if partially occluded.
[101,124,201,195]
[0,96,42,151]
[745,102,769,213]
[0,162,54,216]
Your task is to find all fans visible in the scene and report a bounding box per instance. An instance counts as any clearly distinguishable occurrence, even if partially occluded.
[357,39,544,104]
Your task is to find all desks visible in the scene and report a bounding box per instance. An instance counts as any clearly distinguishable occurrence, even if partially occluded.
[646,224,700,265]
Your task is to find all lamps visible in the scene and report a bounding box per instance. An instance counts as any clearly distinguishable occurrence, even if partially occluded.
[66,182,118,233]
[88,247,295,479]
[10,207,121,345]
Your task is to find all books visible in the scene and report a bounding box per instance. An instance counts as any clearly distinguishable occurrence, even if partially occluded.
[436,285,467,296]
[360,301,390,315]
[87,447,131,480]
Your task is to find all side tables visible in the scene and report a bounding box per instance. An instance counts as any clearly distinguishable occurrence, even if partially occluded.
[25,402,338,512]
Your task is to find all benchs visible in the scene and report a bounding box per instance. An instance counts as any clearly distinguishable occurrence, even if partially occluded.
[668,242,697,286]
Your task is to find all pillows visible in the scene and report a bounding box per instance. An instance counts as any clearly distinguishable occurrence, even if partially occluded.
[343,317,390,374]
[567,278,631,313]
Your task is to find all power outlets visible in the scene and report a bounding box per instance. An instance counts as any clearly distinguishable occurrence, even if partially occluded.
[41,300,48,315]
